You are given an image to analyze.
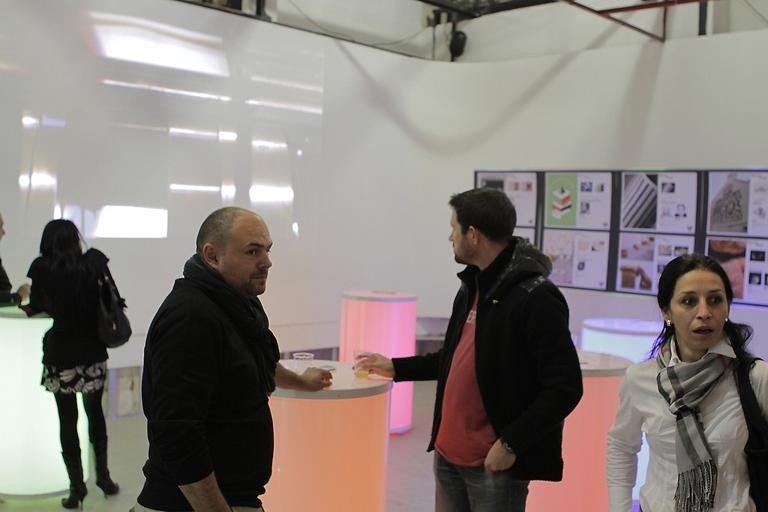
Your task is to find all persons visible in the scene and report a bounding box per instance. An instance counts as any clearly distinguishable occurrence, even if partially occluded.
[128,206,333,512]
[0,214,31,307]
[16,219,119,511]
[352,186,583,512]
[607,254,768,512]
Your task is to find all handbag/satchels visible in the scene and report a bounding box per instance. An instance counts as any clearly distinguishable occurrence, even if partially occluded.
[744,419,768,512]
[94,278,131,350]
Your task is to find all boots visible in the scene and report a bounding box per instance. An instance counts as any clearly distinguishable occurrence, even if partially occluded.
[89,429,119,499]
[60,448,88,509]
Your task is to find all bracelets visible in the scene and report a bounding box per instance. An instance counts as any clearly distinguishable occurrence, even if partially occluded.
[500,437,514,454]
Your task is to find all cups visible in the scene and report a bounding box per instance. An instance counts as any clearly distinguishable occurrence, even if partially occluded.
[293,353,314,374]
[352,350,369,379]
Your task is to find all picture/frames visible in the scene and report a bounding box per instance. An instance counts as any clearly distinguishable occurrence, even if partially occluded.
[615,170,702,234]
[539,227,613,291]
[703,169,768,239]
[475,170,539,228]
[541,168,614,232]
[700,236,768,307]
[614,230,699,299]
